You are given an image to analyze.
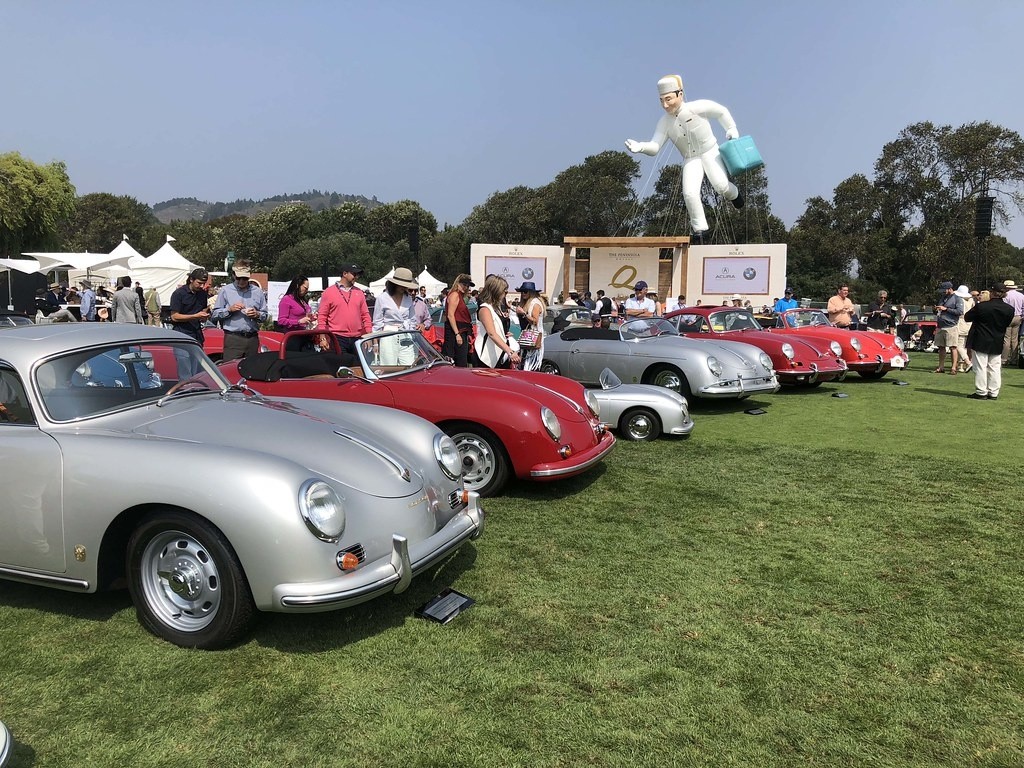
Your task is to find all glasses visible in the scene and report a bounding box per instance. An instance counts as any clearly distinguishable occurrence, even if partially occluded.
[520,290,529,293]
[407,289,418,293]
[350,272,360,277]
[503,290,508,295]
[593,319,600,322]
[302,284,309,292]
[234,275,250,281]
[635,289,643,291]
[785,292,792,295]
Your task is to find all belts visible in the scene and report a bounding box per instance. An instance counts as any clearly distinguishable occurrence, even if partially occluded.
[834,325,849,329]
[225,331,256,336]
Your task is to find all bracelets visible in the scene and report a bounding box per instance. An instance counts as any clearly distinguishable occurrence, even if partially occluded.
[374,344,378,346]
[456,333,459,336]
[509,351,514,355]
[320,338,325,342]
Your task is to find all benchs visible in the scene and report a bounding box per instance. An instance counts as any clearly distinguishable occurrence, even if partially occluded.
[265,330,365,381]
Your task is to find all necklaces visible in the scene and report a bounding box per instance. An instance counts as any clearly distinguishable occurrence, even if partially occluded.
[336,284,351,304]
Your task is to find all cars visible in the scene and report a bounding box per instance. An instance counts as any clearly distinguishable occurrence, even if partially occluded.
[128,325,290,386]
[543,304,597,333]
[897,311,938,351]
[430,304,524,340]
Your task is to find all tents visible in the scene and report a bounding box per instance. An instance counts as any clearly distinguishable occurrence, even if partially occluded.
[67,241,205,307]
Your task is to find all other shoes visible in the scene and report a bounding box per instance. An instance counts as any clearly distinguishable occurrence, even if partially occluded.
[967,393,997,400]
[933,363,972,375]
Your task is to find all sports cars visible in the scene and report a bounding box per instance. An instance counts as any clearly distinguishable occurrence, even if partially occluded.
[0,321,488,653]
[649,304,851,392]
[534,317,783,405]
[585,367,696,443]
[760,308,911,381]
[177,327,620,498]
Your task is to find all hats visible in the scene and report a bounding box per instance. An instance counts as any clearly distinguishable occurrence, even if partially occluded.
[785,287,794,294]
[953,285,972,298]
[47,283,62,291]
[339,262,362,274]
[231,266,251,278]
[515,282,542,293]
[79,279,93,289]
[385,268,419,290]
[1000,280,1019,288]
[592,315,600,321]
[988,282,1009,292]
[937,282,953,294]
[187,268,208,282]
[730,294,743,301]
[633,281,647,290]
[33,289,48,298]
[459,279,475,287]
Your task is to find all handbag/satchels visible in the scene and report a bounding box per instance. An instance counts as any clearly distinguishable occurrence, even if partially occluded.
[518,317,542,349]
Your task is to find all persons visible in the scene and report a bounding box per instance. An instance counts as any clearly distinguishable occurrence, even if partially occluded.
[827,283,856,330]
[170,268,212,380]
[774,287,799,328]
[277,276,318,334]
[722,294,779,315]
[564,281,661,336]
[1001,280,1024,366]
[207,259,267,362]
[318,263,372,371]
[441,273,546,372]
[624,75,744,245]
[918,303,926,320]
[672,295,701,323]
[964,282,1014,401]
[899,304,907,321]
[372,268,447,367]
[865,290,898,336]
[932,285,990,373]
[36,276,162,326]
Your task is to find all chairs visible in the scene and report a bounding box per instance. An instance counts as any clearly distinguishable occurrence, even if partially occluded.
[897,323,937,351]
